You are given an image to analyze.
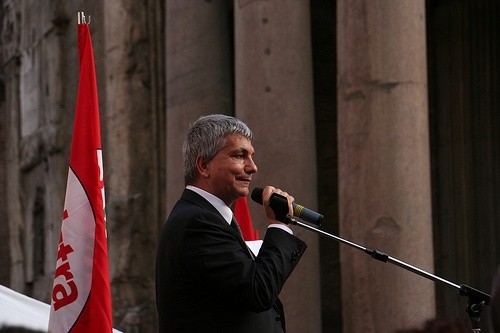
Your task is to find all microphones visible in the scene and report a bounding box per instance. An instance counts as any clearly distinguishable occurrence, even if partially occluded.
[251,186,325,226]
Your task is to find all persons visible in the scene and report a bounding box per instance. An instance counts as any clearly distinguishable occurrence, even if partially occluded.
[154,115,307,333]
[48,23,113,333]
